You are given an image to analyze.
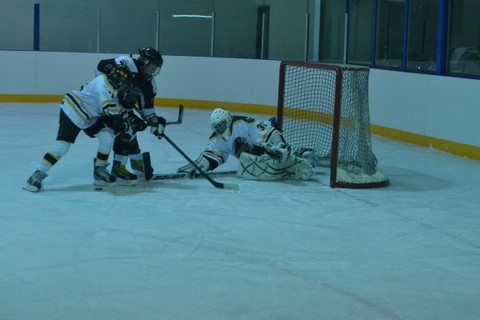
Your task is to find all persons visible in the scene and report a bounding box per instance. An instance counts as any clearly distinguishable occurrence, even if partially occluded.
[177,107,319,179]
[23,65,159,192]
[94,46,166,185]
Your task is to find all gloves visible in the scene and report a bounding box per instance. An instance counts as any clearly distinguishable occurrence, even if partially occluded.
[124,113,146,135]
[118,90,139,110]
[150,116,166,140]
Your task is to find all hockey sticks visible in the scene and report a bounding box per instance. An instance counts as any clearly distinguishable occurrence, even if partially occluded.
[131,103,240,191]
[142,148,238,183]
[129,104,185,128]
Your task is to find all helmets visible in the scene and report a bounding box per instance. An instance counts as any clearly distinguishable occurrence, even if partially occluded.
[108,64,133,92]
[210,108,233,138]
[138,46,163,70]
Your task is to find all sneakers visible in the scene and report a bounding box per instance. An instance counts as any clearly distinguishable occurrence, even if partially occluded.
[93,163,118,187]
[111,160,139,187]
[22,170,48,192]
[130,159,154,177]
